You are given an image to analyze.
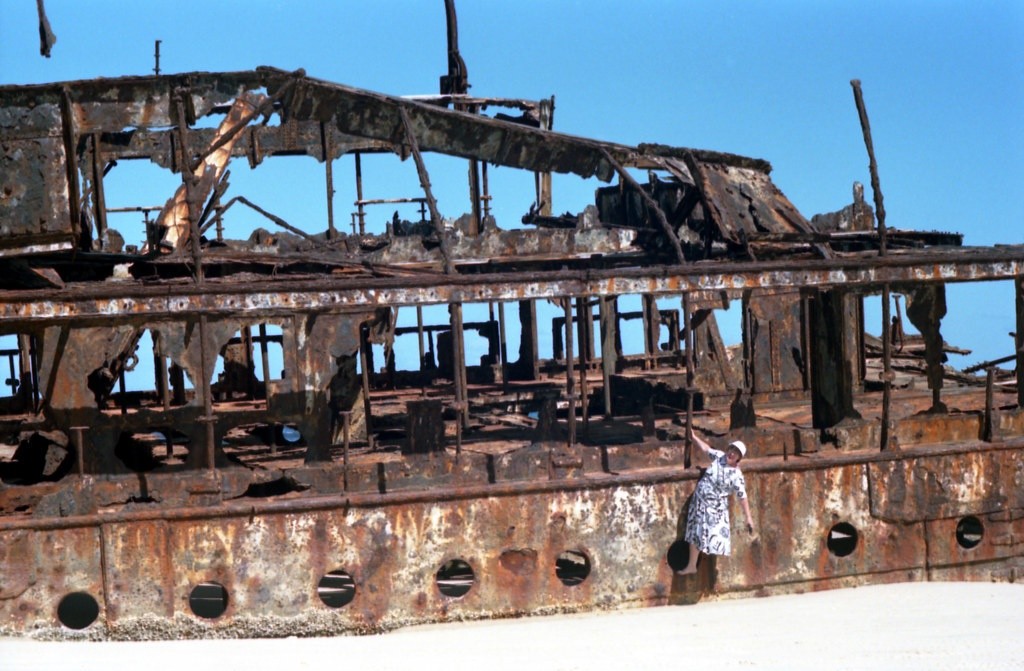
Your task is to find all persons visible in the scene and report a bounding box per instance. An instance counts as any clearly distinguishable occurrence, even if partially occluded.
[674,428,755,576]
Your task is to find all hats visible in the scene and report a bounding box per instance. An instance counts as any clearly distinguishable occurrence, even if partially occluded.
[728,440,747,458]
